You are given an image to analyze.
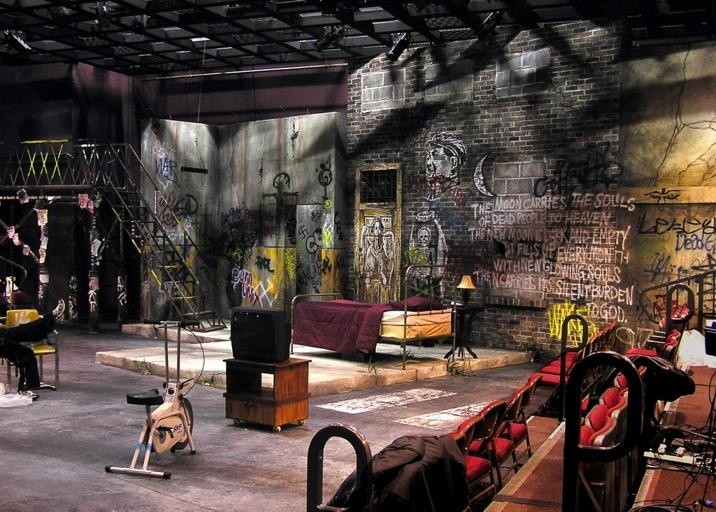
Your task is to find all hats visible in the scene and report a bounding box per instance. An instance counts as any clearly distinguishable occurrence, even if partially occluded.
[425,132,467,164]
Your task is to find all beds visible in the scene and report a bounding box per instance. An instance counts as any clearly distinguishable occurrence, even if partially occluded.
[292,265,455,370]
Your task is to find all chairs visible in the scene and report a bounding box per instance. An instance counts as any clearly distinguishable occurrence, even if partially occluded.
[562,348,695,512]
[644,304,691,365]
[7,309,60,395]
[450,374,543,512]
[521,315,627,427]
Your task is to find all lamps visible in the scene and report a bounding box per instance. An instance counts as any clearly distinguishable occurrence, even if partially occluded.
[313,12,345,52]
[455,274,477,304]
[382,24,412,61]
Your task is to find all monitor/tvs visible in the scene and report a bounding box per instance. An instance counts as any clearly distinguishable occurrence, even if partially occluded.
[230,306,291,361]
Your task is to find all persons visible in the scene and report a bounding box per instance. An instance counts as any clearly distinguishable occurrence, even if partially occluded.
[362,218,387,284]
[406,227,440,280]
[0,298,66,401]
[380,230,395,282]
[423,133,470,202]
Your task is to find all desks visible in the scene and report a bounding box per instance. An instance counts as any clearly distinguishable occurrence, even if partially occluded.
[222,353,312,432]
[444,302,489,361]
